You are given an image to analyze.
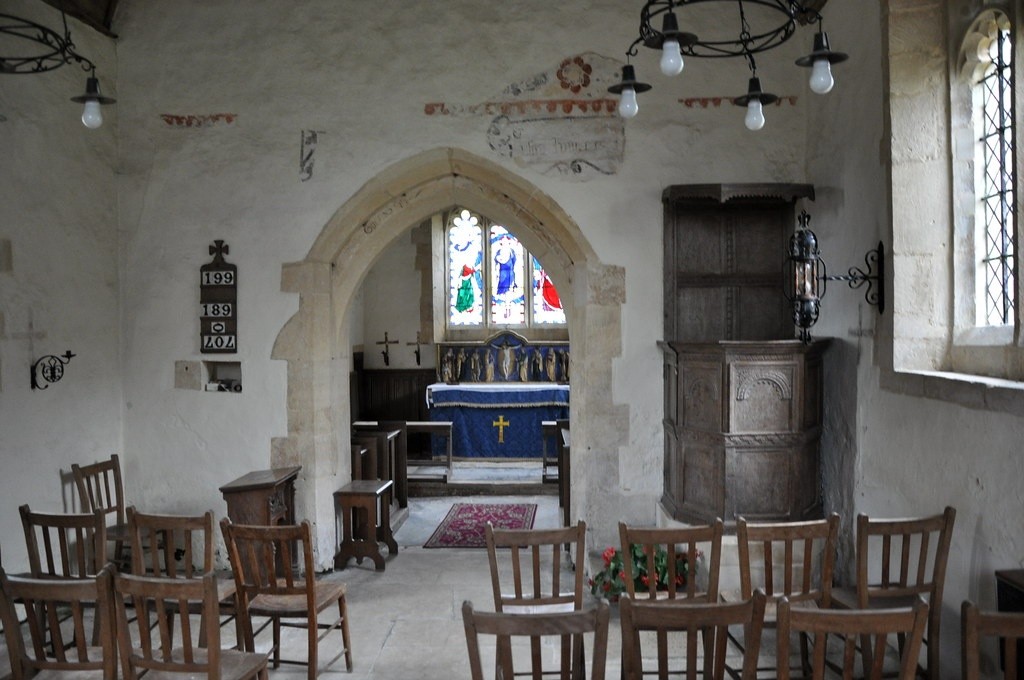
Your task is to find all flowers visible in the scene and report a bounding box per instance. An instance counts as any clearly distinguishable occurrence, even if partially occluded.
[588,538,703,605]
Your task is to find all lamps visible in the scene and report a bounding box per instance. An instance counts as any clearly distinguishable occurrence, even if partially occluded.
[0,0,117,129]
[780,210,884,347]
[609,1,849,132]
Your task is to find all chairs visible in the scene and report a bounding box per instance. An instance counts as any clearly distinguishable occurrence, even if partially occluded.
[776,596,929,680]
[619,587,767,680]
[462,598,609,680]
[19,504,117,658]
[0,566,118,680]
[112,570,270,680]
[125,505,245,652]
[219,516,353,680]
[619,517,727,680]
[72,453,169,608]
[825,506,956,680]
[720,511,841,680]
[484,520,588,680]
[960,600,1024,680]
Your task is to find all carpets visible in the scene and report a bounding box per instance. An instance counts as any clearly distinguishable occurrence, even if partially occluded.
[423,502,538,549]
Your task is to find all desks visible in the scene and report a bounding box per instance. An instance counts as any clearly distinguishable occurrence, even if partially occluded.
[220,464,300,600]
[426,384,569,460]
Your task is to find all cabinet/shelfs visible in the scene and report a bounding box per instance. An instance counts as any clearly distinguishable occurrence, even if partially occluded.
[352,420,454,484]
[542,420,559,483]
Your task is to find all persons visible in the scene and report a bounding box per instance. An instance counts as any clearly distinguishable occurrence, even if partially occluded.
[441,341,570,382]
